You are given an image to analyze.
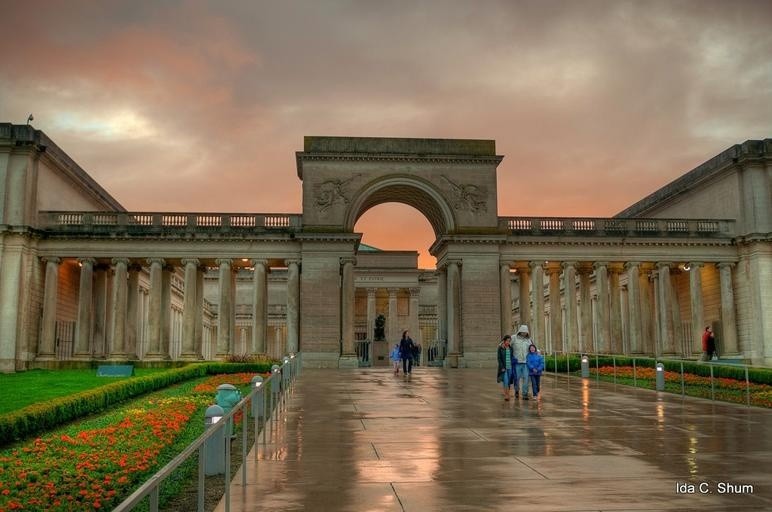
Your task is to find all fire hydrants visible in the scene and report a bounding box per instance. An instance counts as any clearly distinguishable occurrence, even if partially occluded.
[213,382,243,440]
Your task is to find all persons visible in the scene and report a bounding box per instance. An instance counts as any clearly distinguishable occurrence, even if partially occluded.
[702,326,711,362]
[497,335,513,401]
[526,344,544,401]
[707,332,716,360]
[389,331,422,376]
[499,325,540,400]
